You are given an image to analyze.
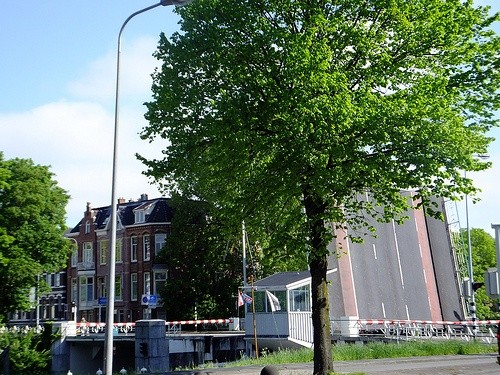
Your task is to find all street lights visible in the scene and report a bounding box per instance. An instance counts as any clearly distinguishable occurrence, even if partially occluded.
[102,0,186,375]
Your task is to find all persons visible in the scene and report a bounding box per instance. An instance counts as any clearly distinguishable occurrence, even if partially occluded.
[81,317,87,336]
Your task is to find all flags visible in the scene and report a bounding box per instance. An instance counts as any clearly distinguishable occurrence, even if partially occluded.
[266,290,281,311]
[238,291,253,308]
